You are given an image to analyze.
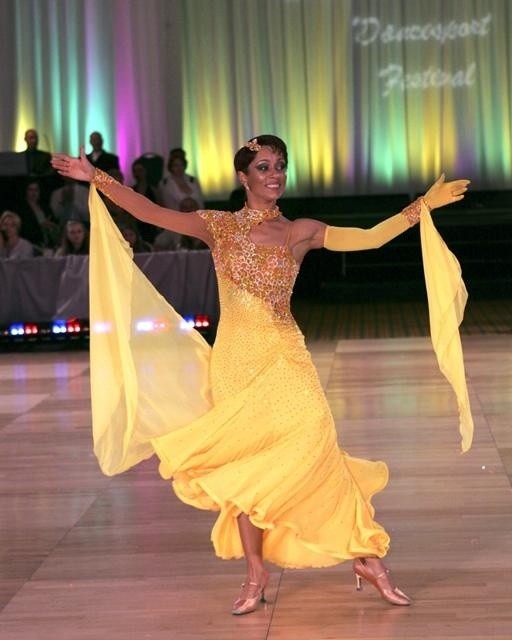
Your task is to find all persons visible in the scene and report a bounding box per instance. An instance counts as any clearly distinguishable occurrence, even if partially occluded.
[2,130,208,258]
[50,135,470,615]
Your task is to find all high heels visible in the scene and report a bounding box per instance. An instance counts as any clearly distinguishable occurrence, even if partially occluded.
[232,568,268,615]
[352,557,414,606]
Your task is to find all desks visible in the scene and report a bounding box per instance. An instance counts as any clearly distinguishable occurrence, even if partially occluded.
[0,249,219,353]
